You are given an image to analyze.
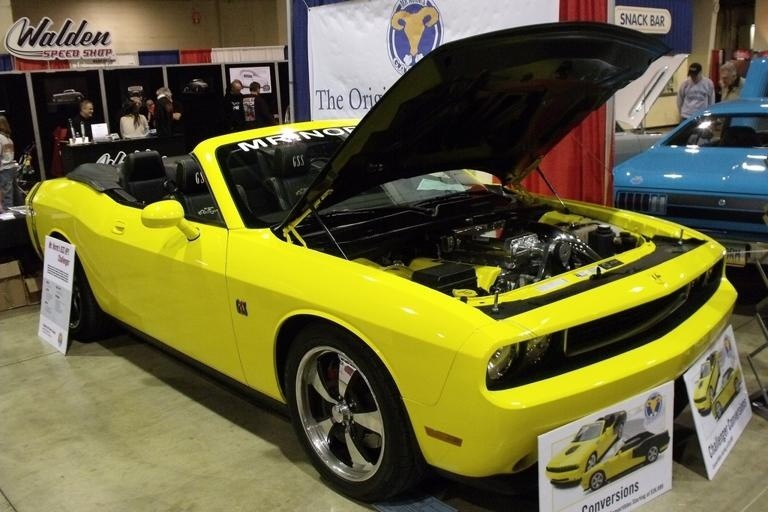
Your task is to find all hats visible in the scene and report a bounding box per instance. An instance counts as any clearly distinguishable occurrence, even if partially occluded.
[687,63,702,77]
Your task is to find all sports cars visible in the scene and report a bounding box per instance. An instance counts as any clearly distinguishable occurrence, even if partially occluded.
[24,19,738,506]
[611,52,768,307]
[579,429,669,492]
[544,410,627,489]
[694,350,742,421]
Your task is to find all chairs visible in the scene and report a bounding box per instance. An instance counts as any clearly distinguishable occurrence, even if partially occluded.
[717,126,758,150]
[121,134,326,234]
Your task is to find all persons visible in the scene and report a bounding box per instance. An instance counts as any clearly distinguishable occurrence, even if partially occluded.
[47,79,275,176]
[0,118,20,219]
[718,62,745,102]
[677,62,716,124]
